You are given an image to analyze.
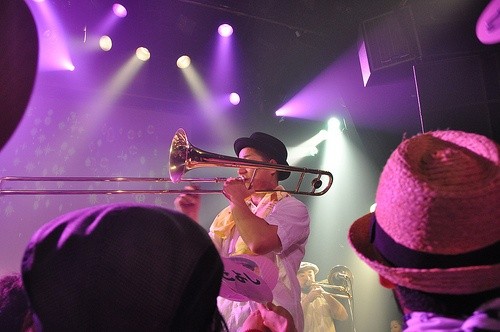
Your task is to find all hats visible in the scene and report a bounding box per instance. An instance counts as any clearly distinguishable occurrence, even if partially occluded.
[21,201,225,332]
[348,129,500,294]
[234,132,291,181]
[297,261,320,276]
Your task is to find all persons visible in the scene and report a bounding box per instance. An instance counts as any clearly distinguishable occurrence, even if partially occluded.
[21,202,224,332]
[349,131,500,332]
[297,261,349,332]
[174,132,311,332]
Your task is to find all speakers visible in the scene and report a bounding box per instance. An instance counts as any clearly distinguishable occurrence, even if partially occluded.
[413,54,494,140]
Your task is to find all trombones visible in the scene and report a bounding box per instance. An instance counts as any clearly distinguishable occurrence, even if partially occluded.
[0,127,334,196]
[305,265,354,300]
